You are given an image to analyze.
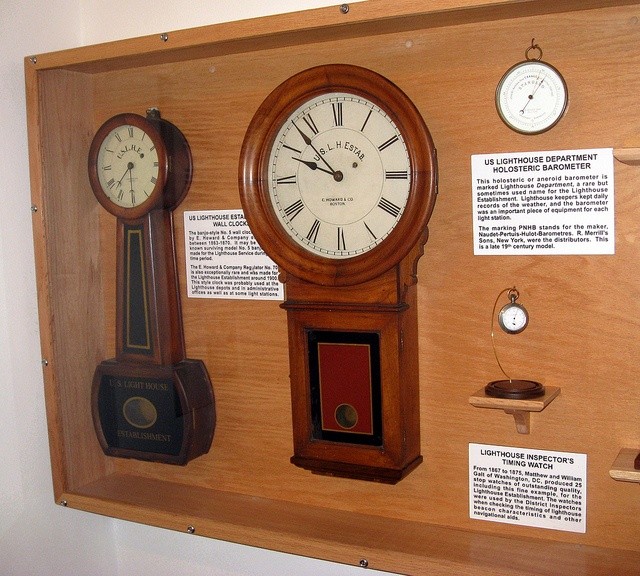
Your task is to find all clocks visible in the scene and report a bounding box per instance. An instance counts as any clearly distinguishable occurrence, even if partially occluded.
[498,303,529,335]
[88,113,216,467]
[237,63,438,486]
[494,60,568,135]
